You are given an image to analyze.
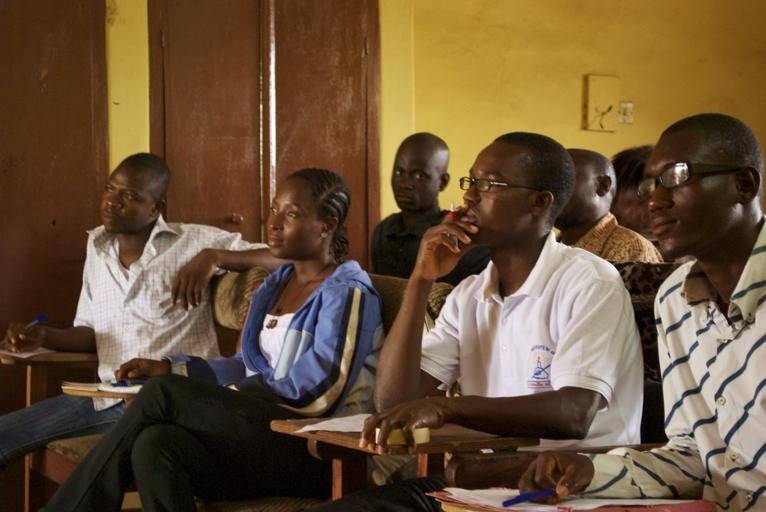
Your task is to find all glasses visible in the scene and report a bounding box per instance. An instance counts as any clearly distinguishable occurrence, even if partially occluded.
[636,160,742,204]
[459,177,540,193]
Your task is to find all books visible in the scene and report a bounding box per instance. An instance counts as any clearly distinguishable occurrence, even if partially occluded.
[57,379,144,396]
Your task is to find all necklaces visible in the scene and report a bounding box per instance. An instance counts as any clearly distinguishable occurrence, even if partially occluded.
[266,257,333,330]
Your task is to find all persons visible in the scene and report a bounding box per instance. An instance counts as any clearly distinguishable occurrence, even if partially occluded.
[554,149,665,265]
[611,146,657,245]
[0,153,291,471]
[314,132,644,512]
[519,112,766,510]
[43,169,384,512]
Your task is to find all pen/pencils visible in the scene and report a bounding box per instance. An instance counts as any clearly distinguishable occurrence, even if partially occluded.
[111,377,147,387]
[502,487,556,506]
[449,201,459,253]
[0,314,47,345]
